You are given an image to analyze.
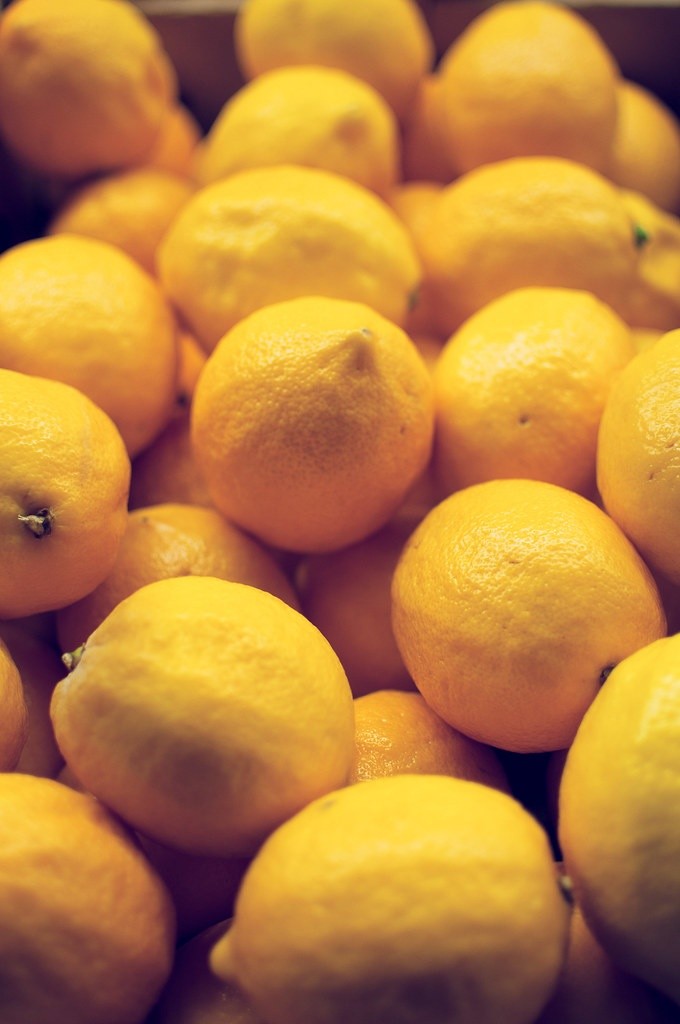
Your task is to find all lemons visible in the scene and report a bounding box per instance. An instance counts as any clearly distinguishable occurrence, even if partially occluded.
[0,0,680,1024]
